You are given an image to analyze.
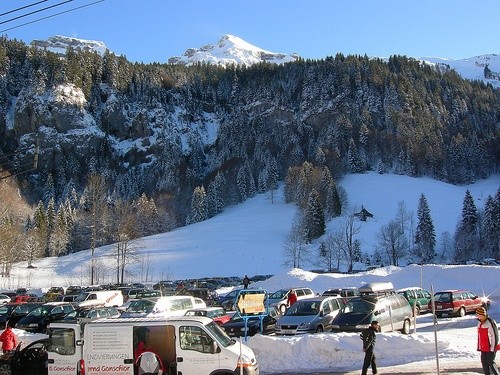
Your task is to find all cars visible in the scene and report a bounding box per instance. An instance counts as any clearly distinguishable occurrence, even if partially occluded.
[0,278,433,336]
[428,290,487,317]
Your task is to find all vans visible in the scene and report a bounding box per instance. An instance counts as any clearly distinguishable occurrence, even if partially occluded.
[0,315,259,374]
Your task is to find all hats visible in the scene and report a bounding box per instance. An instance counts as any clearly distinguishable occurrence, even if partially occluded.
[476,307,486,316]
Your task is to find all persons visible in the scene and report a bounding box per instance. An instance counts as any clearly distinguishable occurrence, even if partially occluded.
[0,326,16,354]
[243,275,250,289]
[475,307,500,375]
[288,289,297,306]
[361,321,379,375]
